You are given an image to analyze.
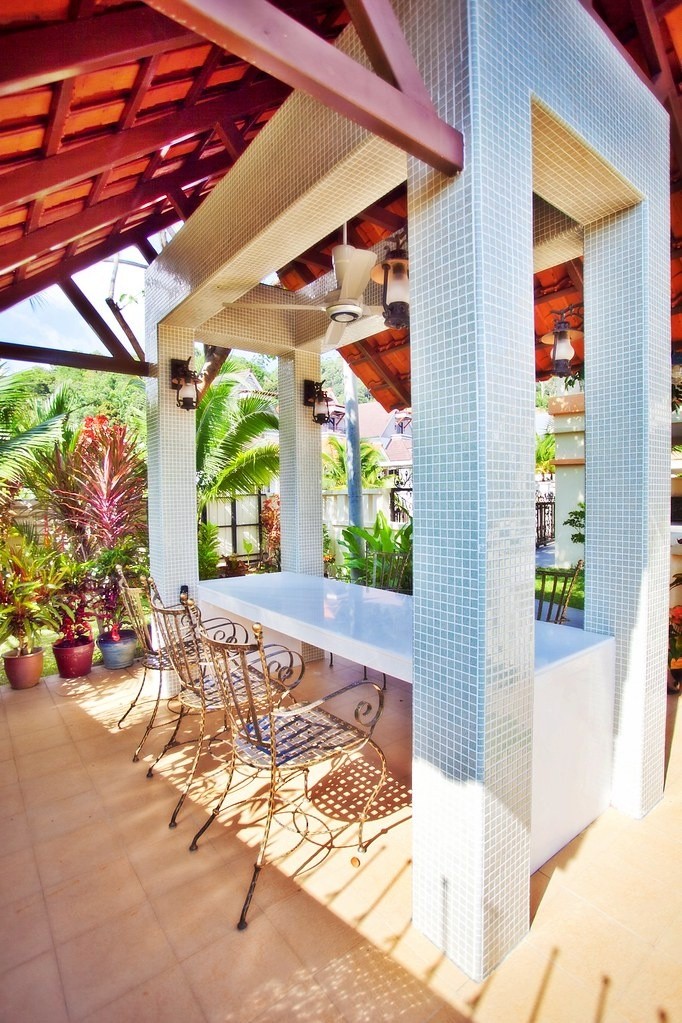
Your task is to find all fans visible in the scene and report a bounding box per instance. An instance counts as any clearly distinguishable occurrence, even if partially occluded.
[222,221,384,346]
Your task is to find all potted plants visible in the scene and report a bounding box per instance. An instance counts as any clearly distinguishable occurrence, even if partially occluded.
[0,534,139,690]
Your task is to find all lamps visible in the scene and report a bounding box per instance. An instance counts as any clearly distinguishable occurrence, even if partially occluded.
[309,380,331,425]
[549,319,575,376]
[381,234,412,331]
[172,365,202,411]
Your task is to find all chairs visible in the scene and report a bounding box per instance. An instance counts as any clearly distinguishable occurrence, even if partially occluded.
[147,578,306,826]
[188,594,386,929]
[115,564,248,761]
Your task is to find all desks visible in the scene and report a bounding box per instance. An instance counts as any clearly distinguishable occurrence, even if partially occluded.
[195,570,618,876]
